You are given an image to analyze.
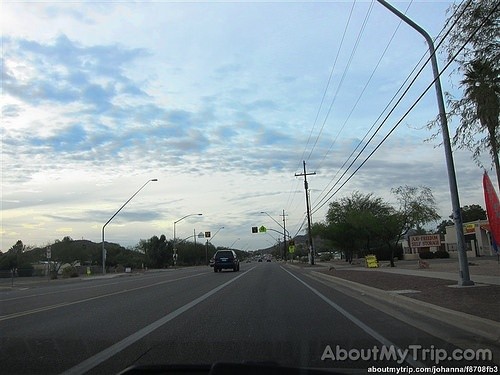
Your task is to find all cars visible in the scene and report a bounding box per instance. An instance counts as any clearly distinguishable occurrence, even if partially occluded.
[246,254,273,263]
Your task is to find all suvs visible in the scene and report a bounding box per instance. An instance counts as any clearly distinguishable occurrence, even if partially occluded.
[214,250,239,272]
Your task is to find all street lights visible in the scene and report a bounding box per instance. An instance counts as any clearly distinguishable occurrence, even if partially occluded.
[229,238,241,250]
[101,178,158,275]
[206,227,225,266]
[172,213,203,267]
[262,212,294,263]
